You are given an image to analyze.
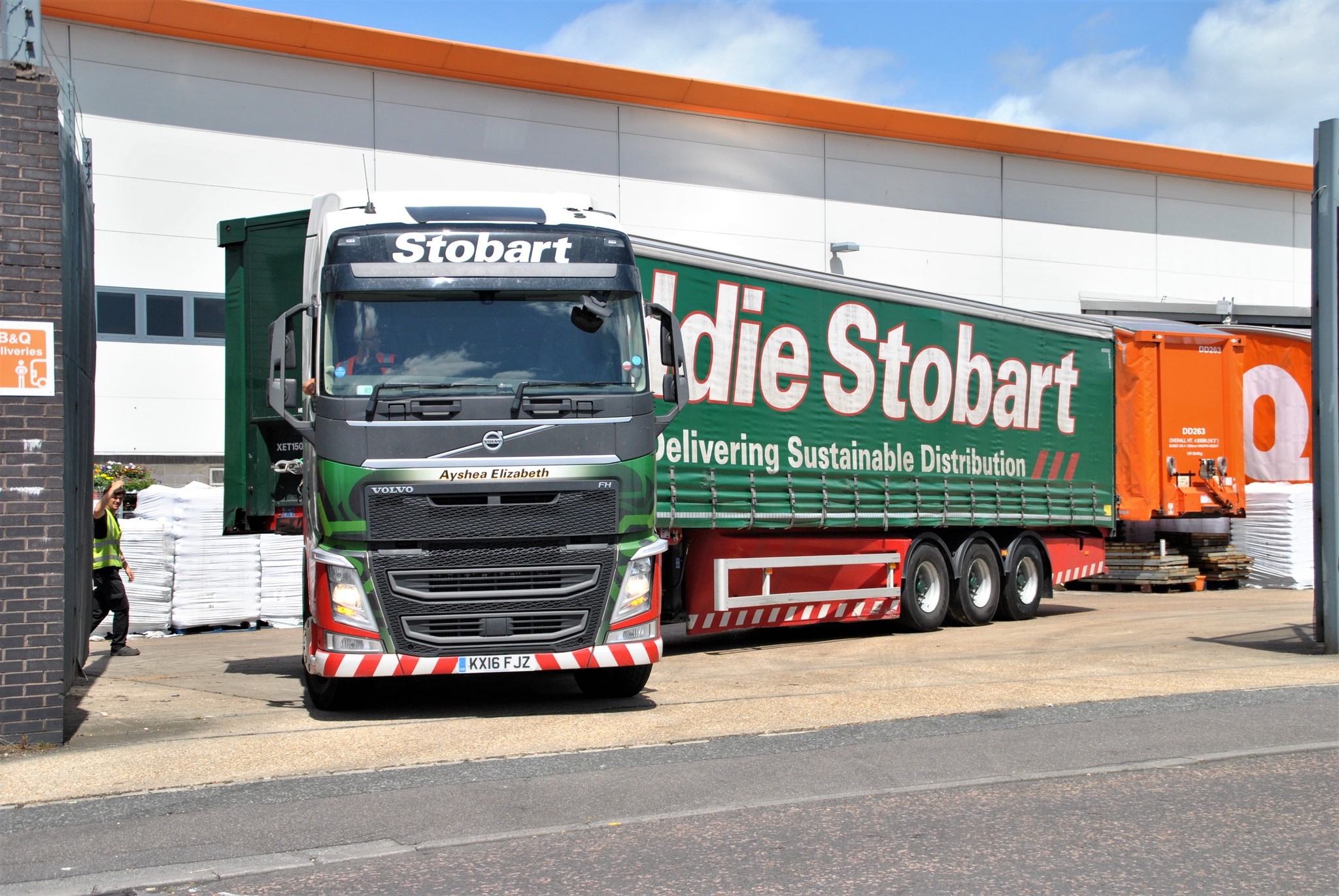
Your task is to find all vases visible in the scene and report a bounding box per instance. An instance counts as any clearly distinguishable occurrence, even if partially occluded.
[123,490,138,511]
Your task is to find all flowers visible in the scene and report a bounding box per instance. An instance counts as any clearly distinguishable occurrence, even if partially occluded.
[93,460,162,495]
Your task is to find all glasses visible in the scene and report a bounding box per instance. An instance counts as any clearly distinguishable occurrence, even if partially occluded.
[361,335,383,342]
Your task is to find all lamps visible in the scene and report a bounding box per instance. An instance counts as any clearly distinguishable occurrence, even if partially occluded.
[830,242,860,253]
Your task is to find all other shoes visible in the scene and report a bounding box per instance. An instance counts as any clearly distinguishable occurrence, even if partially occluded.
[110,645,140,656]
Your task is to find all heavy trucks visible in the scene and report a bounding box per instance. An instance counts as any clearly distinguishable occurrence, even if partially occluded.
[213,192,1125,721]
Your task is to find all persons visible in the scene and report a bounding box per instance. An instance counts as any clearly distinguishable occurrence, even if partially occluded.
[335,325,404,374]
[90,480,140,656]
